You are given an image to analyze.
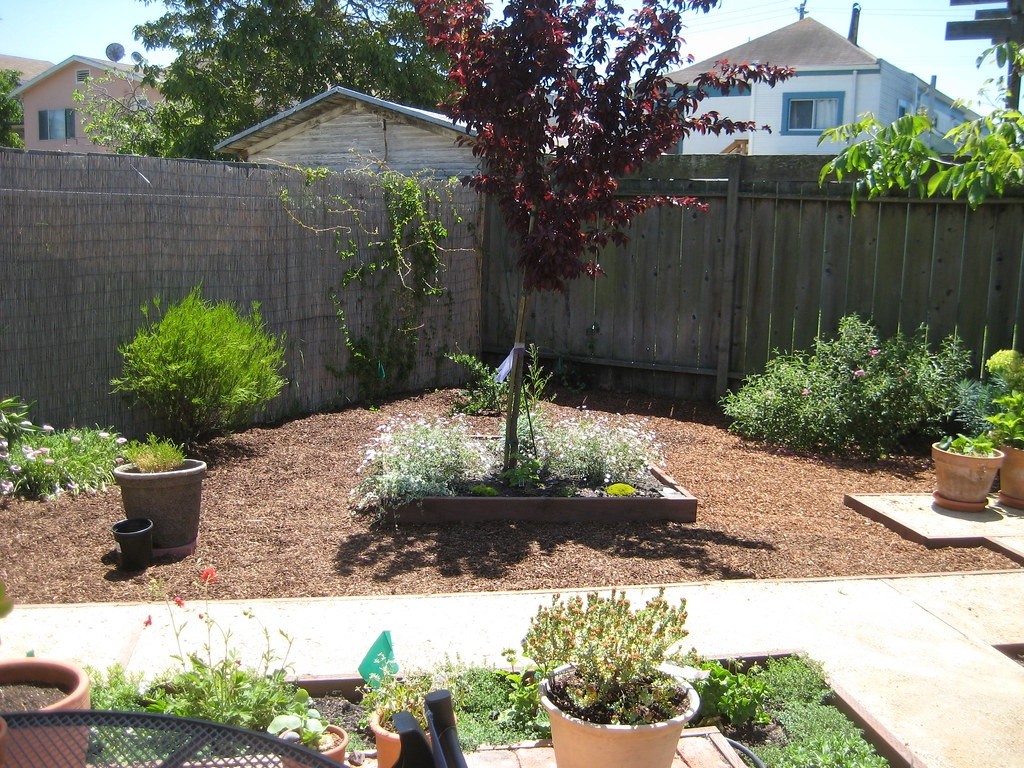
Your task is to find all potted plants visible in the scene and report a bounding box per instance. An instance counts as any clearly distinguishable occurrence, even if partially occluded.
[110,436,208,562]
[266,687,348,768]
[989,391,1024,508]
[932,433,1004,511]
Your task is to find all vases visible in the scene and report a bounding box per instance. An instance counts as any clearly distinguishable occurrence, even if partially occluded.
[113,519,152,575]
[379,454,699,523]
[0,656,89,768]
[539,660,703,768]
[369,706,457,768]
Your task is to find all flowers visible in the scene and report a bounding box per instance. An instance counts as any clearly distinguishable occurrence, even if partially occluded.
[344,397,666,511]
[357,647,435,731]
[526,579,692,715]
